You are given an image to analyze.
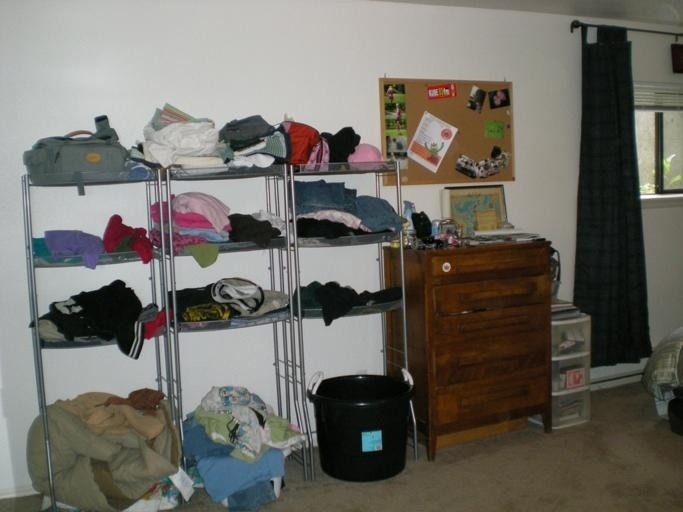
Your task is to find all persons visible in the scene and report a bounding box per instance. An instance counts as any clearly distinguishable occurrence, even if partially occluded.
[395,104,400,122]
[490,94,505,109]
[496,91,509,106]
[391,138,397,153]
[386,86,394,103]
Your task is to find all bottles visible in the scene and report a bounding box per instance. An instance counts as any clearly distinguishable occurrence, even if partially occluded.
[432,219,456,236]
[391,200,417,249]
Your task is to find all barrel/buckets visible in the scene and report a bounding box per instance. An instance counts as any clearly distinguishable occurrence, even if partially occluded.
[309,369,413,483]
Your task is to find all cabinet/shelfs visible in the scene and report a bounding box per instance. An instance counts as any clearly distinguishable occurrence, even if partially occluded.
[527,314,592,431]
[21,160,418,511]
[380,239,554,460]
